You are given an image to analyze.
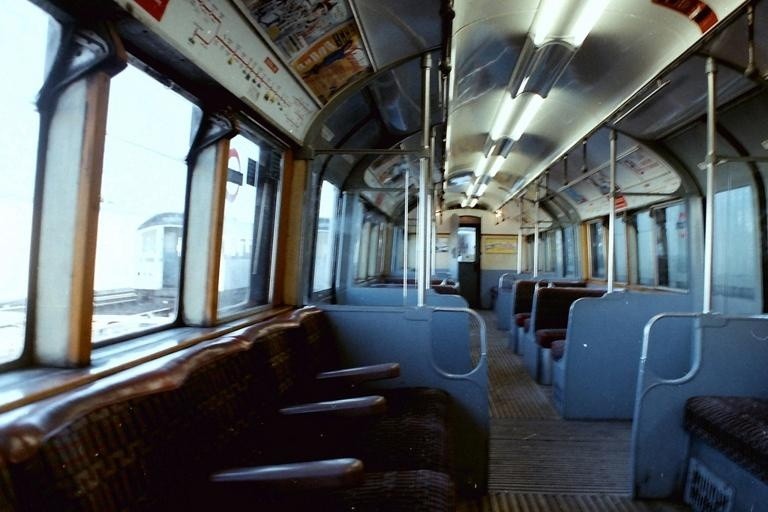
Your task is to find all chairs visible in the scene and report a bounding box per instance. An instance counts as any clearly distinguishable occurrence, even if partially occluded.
[0,274,479,511]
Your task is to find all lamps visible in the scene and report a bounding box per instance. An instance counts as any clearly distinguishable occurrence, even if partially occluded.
[458,0,610,209]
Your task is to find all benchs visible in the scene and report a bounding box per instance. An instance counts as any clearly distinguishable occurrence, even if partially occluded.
[488,271,767,511]
